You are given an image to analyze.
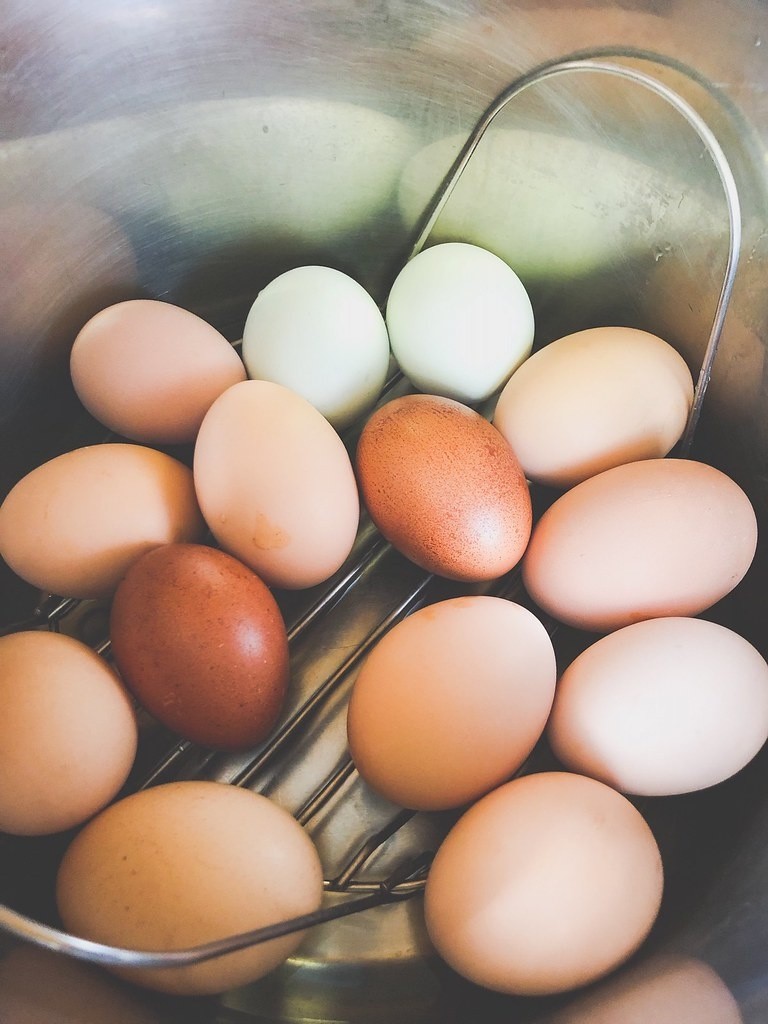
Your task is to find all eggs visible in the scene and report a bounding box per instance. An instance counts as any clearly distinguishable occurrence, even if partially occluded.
[0,238,768,994]
[540,954,746,1024]
[0,100,766,425]
